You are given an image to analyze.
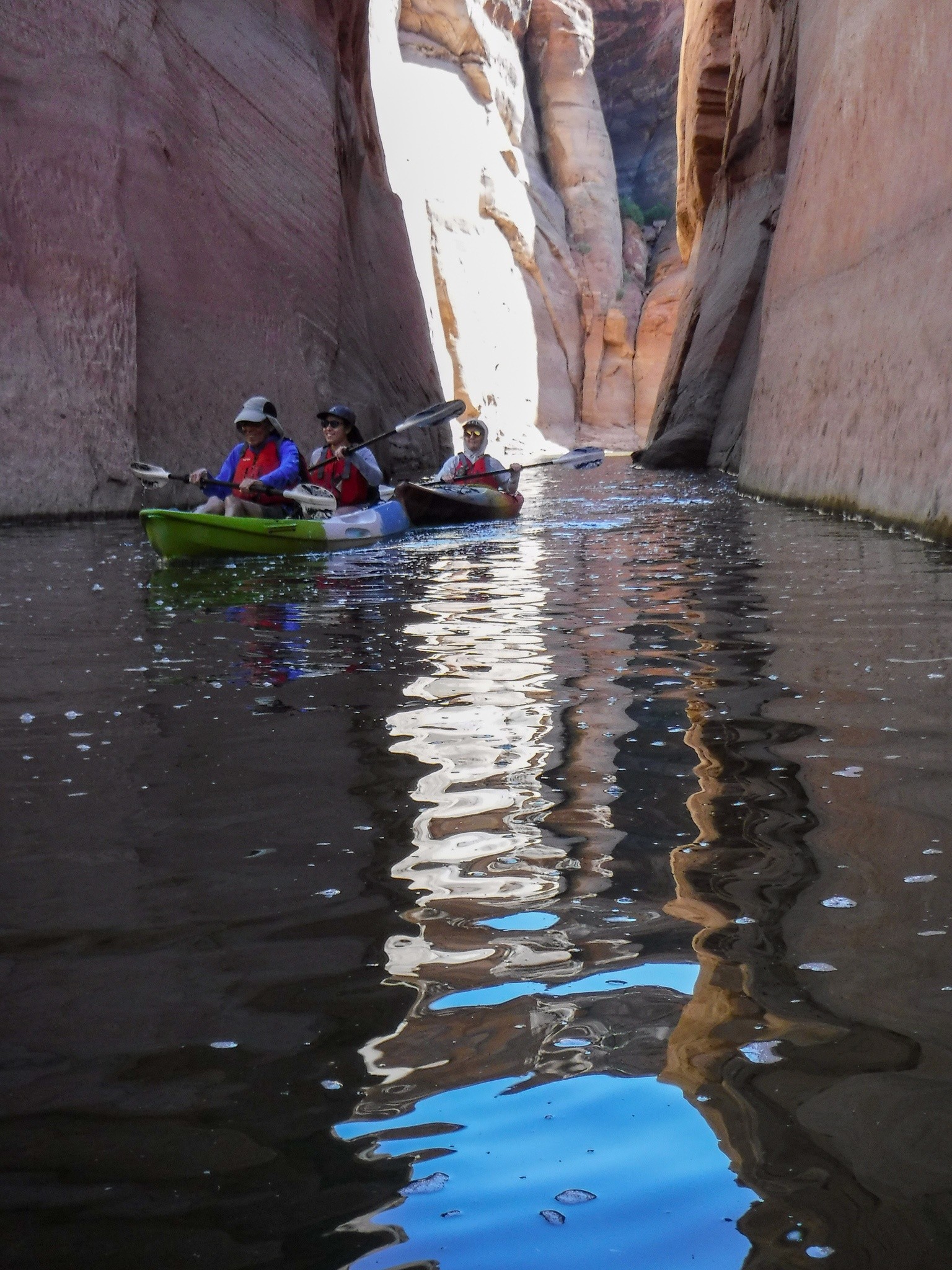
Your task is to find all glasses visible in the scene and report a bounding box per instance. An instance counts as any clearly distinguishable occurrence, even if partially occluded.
[321,419,345,430]
[463,430,483,437]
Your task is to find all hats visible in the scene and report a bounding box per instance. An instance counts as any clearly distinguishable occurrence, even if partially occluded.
[462,421,483,429]
[317,405,357,429]
[235,397,284,438]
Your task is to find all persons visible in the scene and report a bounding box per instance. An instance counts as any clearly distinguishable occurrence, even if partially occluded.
[300,405,384,525]
[432,419,523,496]
[186,395,308,525]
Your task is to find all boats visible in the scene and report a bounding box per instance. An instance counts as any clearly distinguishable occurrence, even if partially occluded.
[139,500,411,558]
[394,478,524,526]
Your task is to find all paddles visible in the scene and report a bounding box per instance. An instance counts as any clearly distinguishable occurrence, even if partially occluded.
[127,460,339,521]
[377,446,605,503]
[309,398,466,474]
